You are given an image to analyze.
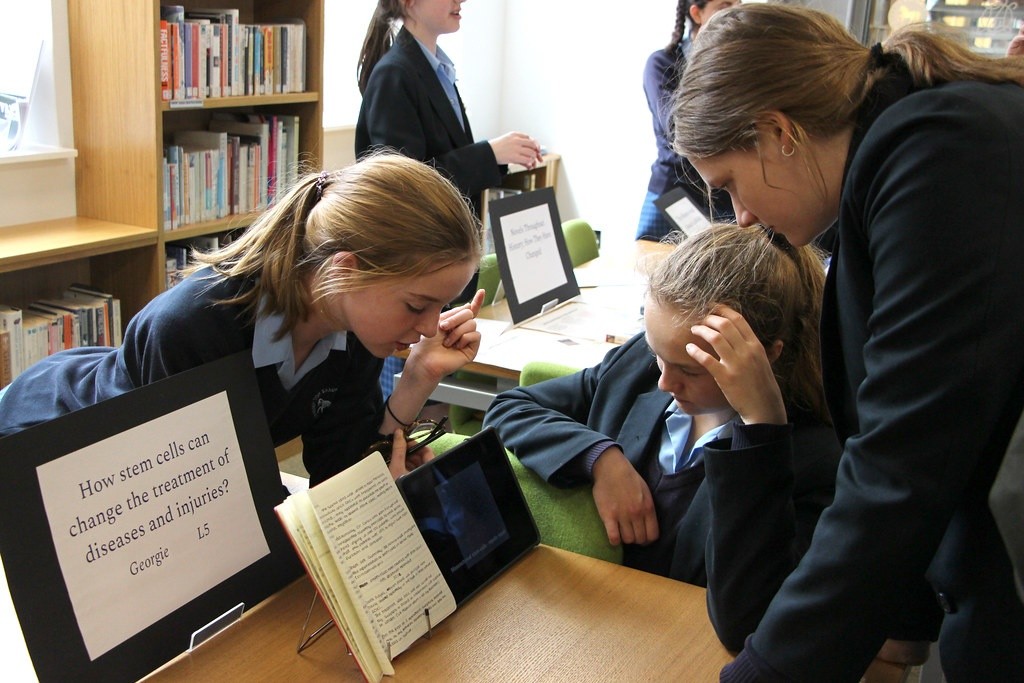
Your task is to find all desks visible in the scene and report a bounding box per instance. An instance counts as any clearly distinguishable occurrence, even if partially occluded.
[139,471,912,683]
[392,240,678,440]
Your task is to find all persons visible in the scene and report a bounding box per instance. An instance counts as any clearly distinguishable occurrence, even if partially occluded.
[0,147,488,502]
[354,0,543,312]
[482,224,842,660]
[636,0,742,243]
[669,0,1024,683]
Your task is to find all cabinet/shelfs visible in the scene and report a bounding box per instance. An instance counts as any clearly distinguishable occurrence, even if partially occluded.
[0,0,324,388]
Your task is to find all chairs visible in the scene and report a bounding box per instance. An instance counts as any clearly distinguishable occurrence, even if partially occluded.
[409,220,622,563]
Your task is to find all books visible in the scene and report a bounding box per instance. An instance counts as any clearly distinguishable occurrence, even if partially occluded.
[275,451,457,683]
[160,6,307,100]
[162,113,299,290]
[0,282,122,390]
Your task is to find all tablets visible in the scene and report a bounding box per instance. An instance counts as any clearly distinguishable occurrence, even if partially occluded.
[393,424,541,610]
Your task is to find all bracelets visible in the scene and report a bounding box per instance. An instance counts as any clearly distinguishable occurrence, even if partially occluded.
[387,395,414,427]
